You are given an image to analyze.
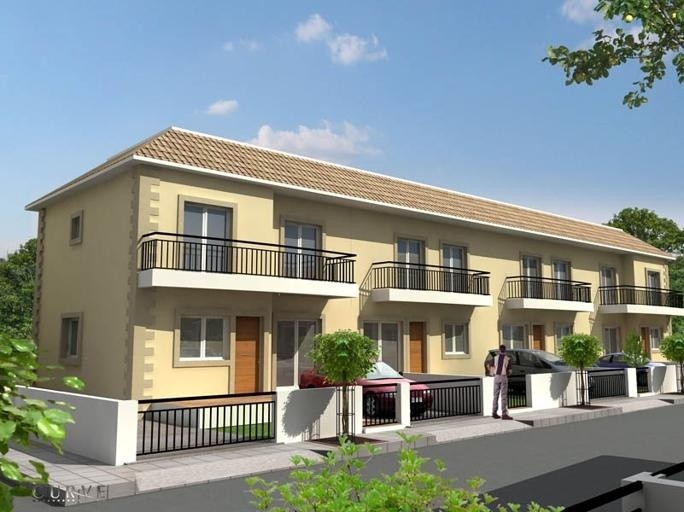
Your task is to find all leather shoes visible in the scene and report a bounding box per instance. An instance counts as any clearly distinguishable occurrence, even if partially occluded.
[502,414,512,420]
[493,413,500,418]
